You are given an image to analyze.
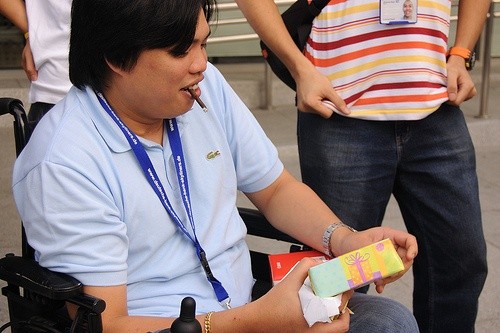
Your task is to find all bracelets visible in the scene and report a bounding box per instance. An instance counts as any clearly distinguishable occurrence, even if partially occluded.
[323,222,359,259]
[24,31,29,39]
[204,311,214,333]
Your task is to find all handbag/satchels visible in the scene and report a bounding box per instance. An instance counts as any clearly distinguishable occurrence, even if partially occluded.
[260,0,331,92]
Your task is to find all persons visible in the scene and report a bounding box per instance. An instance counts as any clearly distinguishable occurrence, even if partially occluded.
[0,0,74,123]
[234,0,491,333]
[400,0,416,22]
[11,0,420,333]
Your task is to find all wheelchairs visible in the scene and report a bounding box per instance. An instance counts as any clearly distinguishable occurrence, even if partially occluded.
[0,97,314,333]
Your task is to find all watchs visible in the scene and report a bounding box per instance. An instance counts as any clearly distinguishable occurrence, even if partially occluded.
[446,45,476,70]
